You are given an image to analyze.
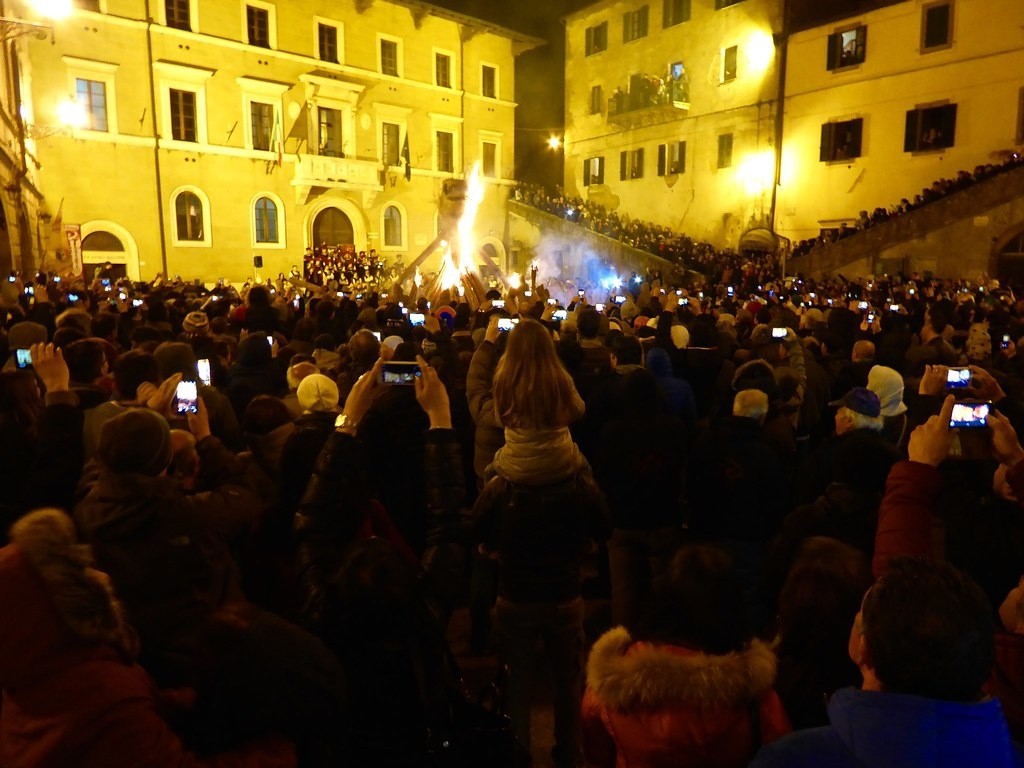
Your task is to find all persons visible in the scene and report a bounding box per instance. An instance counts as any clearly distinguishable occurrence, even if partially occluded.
[0,154,1024,768]
[613,68,688,114]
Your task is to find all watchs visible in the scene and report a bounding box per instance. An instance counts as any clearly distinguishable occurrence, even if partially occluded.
[335,414,358,429]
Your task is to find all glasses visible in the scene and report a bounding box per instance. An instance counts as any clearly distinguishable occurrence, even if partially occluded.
[101,356,108,367]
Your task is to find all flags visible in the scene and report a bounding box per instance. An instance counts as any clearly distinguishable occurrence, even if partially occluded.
[402,136,411,180]
[273,115,284,167]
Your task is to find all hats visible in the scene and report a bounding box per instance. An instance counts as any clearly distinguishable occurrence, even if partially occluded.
[9,322,47,349]
[621,301,638,318]
[828,387,881,416]
[130,326,161,346]
[356,307,376,326]
[392,343,429,363]
[182,311,209,334]
[97,409,170,476]
[297,373,339,413]
[806,308,823,322]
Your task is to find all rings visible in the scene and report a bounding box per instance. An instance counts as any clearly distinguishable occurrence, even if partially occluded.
[933,369,938,372]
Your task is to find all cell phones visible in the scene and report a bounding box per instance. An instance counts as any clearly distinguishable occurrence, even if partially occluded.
[10,248,1021,381]
[376,361,428,385]
[949,398,993,426]
[177,381,198,414]
[945,367,972,390]
